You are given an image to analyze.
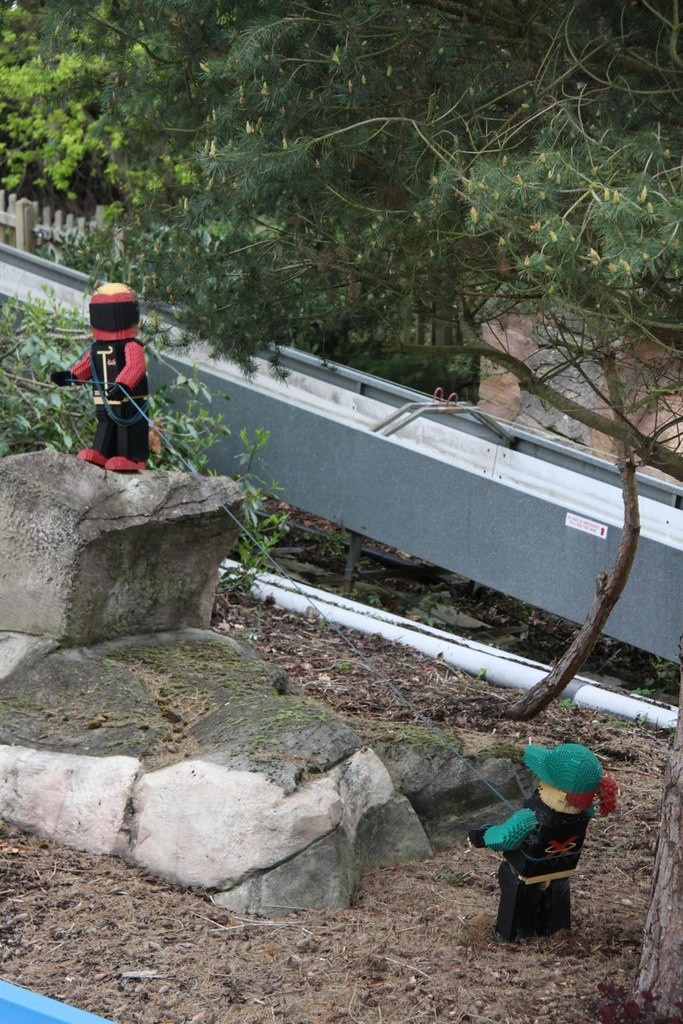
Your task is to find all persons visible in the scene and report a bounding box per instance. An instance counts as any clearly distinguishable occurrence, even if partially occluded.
[51,282,150,470]
[465,742,618,943]
